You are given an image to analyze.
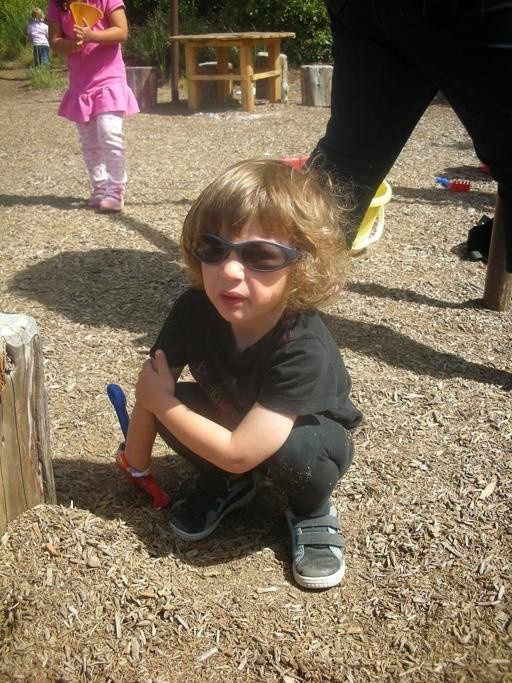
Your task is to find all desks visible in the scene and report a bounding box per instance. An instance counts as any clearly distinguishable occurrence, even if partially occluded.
[169,30,296,113]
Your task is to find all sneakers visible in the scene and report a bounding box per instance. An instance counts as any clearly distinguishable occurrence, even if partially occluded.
[166,476,258,540]
[88,181,108,205]
[285,503,345,590]
[100,184,125,211]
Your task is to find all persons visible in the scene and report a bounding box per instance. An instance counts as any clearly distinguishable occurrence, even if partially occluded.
[46,0,143,213]
[28,5,53,67]
[117,154,363,591]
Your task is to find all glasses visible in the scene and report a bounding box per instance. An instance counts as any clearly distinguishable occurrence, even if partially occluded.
[191,230,301,273]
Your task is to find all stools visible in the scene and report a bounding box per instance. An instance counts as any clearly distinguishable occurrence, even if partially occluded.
[122,64,159,112]
[197,50,334,106]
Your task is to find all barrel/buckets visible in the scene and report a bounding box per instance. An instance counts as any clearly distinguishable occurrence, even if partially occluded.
[349,180,394,255]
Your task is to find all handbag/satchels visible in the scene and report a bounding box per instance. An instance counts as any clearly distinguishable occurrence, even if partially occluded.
[464,215,494,266]
